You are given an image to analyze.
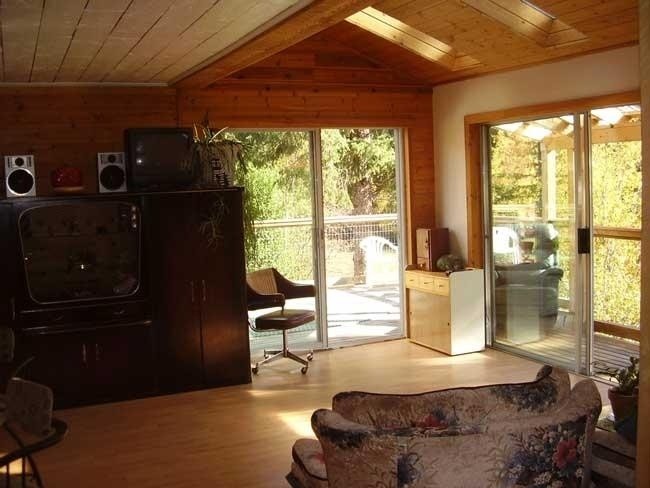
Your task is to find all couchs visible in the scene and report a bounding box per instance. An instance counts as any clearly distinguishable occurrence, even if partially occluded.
[284,364,603,487]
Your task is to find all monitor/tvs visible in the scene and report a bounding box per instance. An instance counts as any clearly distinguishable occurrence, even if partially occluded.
[127,127,193,192]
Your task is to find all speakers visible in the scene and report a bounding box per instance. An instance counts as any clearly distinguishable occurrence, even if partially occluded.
[4,155,37,197]
[97,152,128,193]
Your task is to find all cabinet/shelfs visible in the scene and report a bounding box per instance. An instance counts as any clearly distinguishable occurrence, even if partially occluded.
[415,227,449,272]
[18,320,152,415]
[140,184,251,395]
[403,263,485,356]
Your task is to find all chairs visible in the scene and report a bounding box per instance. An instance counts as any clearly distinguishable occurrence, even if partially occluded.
[246,267,317,375]
[0,324,70,488]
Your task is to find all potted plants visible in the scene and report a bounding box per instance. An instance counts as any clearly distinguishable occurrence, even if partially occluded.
[188,122,249,188]
[590,356,639,433]
